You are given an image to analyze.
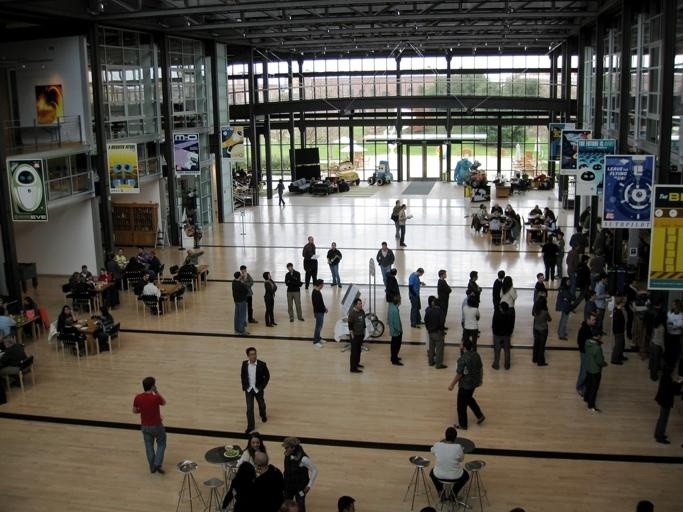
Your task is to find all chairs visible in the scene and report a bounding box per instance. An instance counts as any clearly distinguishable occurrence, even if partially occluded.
[313,342,322,348]
[331,282,335,286]
[319,339,327,344]
[338,284,341,288]
[239,331,249,335]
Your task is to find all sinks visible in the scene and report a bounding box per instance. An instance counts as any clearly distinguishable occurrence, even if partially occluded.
[290,177,339,196]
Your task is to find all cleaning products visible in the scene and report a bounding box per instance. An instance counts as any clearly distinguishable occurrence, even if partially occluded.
[363,258,383,339]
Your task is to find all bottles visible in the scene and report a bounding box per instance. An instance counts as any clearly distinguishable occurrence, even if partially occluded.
[280,437,300,447]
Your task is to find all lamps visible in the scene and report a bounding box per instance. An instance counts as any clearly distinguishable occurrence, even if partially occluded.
[472,214,559,245]
[511,176,554,194]
[0,264,208,397]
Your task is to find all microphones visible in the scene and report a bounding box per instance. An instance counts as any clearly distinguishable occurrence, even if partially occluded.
[176,460,224,511]
[403,457,490,512]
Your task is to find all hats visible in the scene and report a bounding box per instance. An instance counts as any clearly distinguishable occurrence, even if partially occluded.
[331,159,395,187]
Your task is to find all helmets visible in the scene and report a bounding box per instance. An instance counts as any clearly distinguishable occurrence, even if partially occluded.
[223,450,239,458]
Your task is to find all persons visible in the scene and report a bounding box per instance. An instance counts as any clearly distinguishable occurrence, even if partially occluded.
[426,299,448,370]
[232,162,254,191]
[223,460,256,510]
[263,271,279,327]
[384,268,401,301]
[584,329,609,414]
[397,204,413,247]
[327,242,343,288]
[338,496,356,512]
[461,169,683,414]
[377,241,394,285]
[235,431,267,473]
[391,200,401,240]
[637,500,654,512]
[231,271,252,336]
[235,452,282,512]
[281,437,317,512]
[1,244,208,403]
[284,263,306,323]
[240,346,270,433]
[408,268,426,326]
[311,279,328,347]
[437,268,452,332]
[425,296,436,327]
[430,427,470,503]
[652,358,677,444]
[134,375,168,476]
[302,236,318,290]
[274,178,287,206]
[575,313,596,400]
[388,295,404,366]
[447,339,487,430]
[239,265,259,324]
[185,190,198,223]
[347,297,367,373]
[310,170,350,195]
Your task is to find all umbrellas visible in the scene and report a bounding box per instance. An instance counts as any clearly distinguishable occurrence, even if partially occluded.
[391,357,401,360]
[453,422,467,430]
[349,368,361,372]
[265,323,273,327]
[261,415,266,422]
[248,319,257,323]
[298,317,304,321]
[476,416,485,424]
[289,318,294,322]
[392,360,403,365]
[429,361,435,366]
[270,321,276,325]
[610,359,623,365]
[356,364,363,367]
[151,467,156,473]
[656,437,670,444]
[537,361,548,366]
[435,364,447,369]
[153,464,164,473]
[244,427,254,432]
[619,356,627,361]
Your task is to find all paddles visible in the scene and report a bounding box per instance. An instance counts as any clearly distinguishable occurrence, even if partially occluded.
[402,180,435,195]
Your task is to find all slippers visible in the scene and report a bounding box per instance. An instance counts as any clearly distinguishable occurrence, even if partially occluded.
[462,352,482,381]
